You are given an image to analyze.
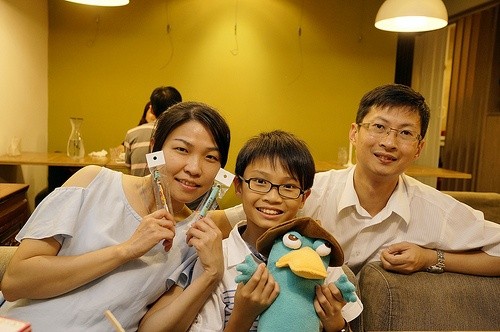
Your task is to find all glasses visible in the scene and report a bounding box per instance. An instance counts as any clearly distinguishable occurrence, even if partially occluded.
[358,122,424,145]
[238,175,305,199]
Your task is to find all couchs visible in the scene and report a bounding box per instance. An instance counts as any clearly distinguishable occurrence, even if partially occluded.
[358,192,500,332]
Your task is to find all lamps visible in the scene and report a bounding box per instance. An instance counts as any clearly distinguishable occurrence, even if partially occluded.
[375,0,448,33]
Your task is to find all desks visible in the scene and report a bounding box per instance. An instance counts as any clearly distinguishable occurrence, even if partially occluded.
[0,153,117,182]
[315,167,472,179]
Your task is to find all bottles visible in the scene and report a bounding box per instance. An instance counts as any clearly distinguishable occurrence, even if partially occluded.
[66,117,85,158]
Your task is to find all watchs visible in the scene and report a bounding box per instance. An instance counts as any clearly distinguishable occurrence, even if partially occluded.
[426,249,445,274]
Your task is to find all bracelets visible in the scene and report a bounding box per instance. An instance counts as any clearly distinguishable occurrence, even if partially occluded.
[322,319,349,332]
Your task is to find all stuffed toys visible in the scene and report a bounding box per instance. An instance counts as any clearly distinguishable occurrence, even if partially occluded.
[234,216,357,332]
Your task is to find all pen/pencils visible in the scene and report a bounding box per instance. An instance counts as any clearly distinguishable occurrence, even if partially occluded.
[197,184,220,220]
[154,167,169,214]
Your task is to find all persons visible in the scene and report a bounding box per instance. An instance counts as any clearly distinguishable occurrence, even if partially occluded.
[187,130,363,332]
[196,84,500,282]
[124,86,182,177]
[0,101,231,332]
